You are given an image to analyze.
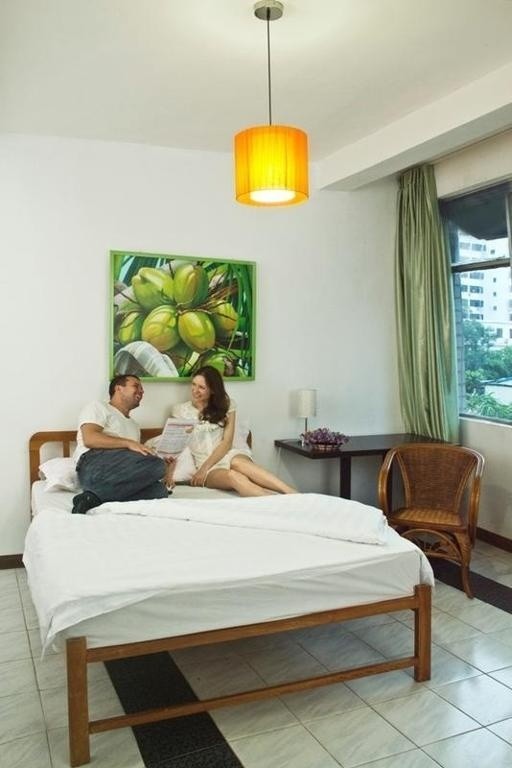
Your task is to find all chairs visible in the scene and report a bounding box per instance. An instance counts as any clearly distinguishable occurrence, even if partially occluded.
[377,441,485,600]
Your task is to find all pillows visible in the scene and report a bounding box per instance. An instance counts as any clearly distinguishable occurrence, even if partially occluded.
[142,428,252,484]
[38,457,82,493]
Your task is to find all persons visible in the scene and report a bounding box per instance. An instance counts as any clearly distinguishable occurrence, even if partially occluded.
[70,373,175,513]
[164,366,298,497]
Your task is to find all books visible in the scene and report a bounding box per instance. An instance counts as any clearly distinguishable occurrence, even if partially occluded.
[156,417,197,462]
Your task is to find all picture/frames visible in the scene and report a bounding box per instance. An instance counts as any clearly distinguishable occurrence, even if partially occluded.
[109,249,257,386]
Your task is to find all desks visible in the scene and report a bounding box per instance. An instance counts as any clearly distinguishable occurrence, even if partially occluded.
[274,433,460,515]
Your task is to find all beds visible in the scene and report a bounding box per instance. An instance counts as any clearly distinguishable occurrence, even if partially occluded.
[27,427,434,768]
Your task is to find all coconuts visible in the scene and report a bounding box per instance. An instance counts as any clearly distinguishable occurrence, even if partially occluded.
[118,263,245,377]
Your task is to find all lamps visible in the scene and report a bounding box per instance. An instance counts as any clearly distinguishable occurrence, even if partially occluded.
[296,388,318,442]
[233,0,311,207]
